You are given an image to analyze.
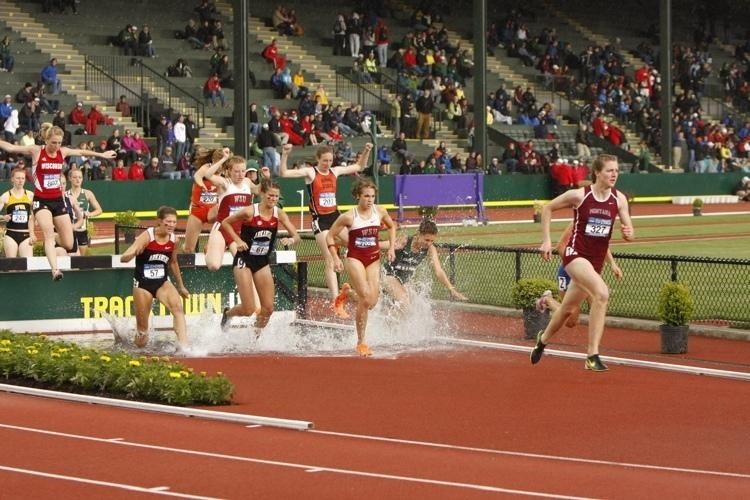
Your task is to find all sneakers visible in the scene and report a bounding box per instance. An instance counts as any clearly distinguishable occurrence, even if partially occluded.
[585,355,608,371]
[530,330,547,363]
[537,290,552,311]
[53,270,64,281]
[333,283,350,318]
[356,344,371,356]
[222,307,229,324]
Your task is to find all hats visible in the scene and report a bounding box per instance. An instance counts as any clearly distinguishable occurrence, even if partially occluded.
[246,159,258,173]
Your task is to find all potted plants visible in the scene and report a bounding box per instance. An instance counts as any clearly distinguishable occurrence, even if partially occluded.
[658,280,695,354]
[532,198,544,224]
[690,198,703,217]
[114,208,144,242]
[87,222,94,246]
[512,276,562,339]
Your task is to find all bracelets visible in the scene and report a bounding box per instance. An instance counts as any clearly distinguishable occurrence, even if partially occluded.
[447,286,455,291]
[289,238,295,244]
[327,244,336,248]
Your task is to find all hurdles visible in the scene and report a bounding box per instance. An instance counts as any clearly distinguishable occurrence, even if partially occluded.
[1,251,310,323]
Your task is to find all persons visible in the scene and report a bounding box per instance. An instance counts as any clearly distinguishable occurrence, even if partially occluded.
[120,207,190,352]
[0,126,117,280]
[536,218,620,328]
[530,154,634,371]
[0,0,750,207]
[203,148,270,272]
[176,148,232,254]
[64,169,102,256]
[335,220,461,319]
[220,178,300,338]
[279,144,373,312]
[0,167,37,257]
[325,178,395,355]
